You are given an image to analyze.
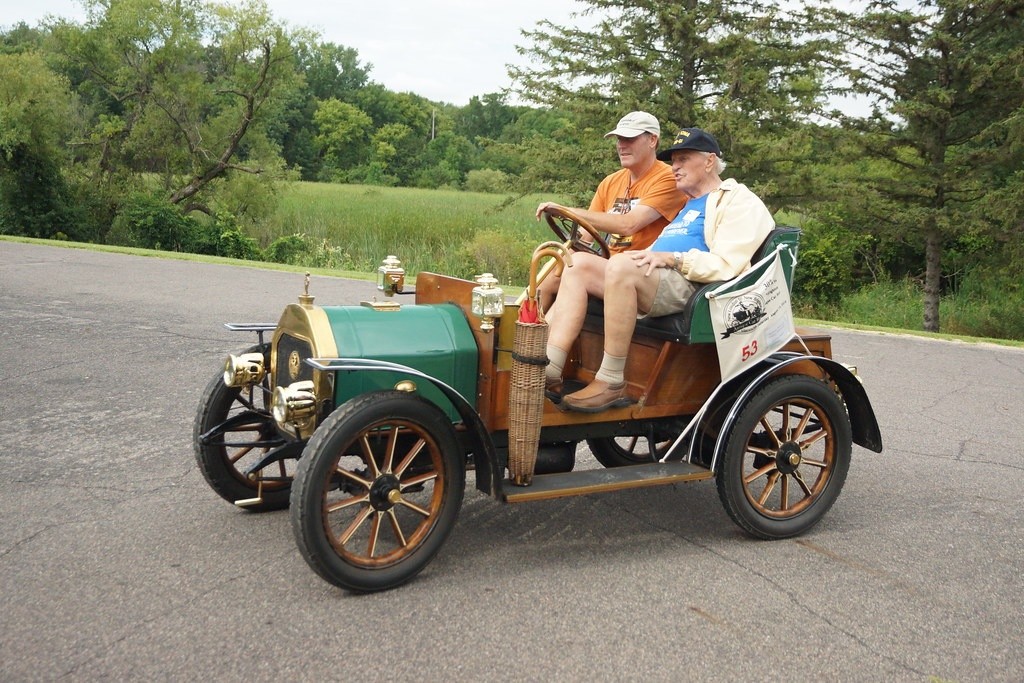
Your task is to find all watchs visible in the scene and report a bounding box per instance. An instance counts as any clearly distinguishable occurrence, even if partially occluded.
[673,252,681,270]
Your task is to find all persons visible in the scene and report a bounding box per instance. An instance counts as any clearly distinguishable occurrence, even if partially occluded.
[543,127,775,413]
[535,111,690,313]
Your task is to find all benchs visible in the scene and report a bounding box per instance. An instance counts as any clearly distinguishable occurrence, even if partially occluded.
[580,225,803,346]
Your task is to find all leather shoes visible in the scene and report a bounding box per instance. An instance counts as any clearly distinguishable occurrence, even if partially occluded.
[545,375,562,403]
[563,380,631,411]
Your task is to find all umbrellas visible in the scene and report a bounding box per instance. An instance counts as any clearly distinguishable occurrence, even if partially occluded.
[519,241,573,324]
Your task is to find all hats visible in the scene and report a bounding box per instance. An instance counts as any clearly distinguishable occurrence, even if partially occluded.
[604,111,661,142]
[653,127,723,161]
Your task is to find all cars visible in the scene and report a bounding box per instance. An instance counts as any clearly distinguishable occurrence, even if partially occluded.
[191,198,888,595]
